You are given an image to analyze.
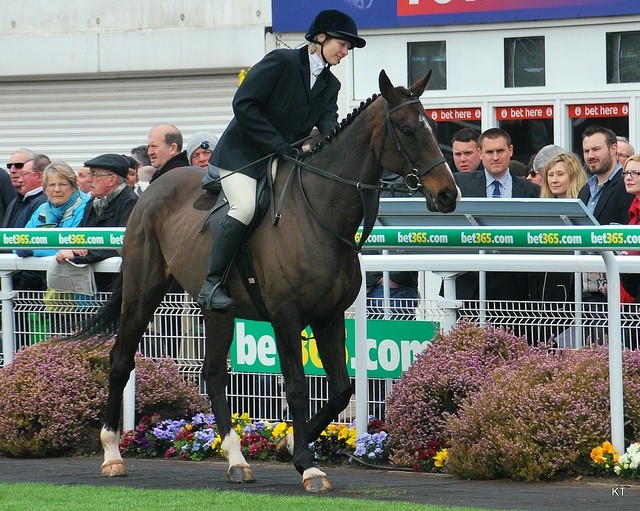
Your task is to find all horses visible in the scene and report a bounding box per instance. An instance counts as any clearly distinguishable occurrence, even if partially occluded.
[37,68,458,495]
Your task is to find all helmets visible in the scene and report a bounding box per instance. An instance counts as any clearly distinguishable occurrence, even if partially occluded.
[305,10,366,68]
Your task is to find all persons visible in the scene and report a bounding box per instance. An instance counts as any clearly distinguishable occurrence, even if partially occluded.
[527,155,548,187]
[9,156,49,229]
[620,154,640,306]
[616,136,633,167]
[611,224,640,300]
[12,163,88,256]
[130,146,152,166]
[450,127,542,197]
[148,123,190,185]
[1,149,35,227]
[119,154,141,198]
[452,127,482,173]
[575,125,631,342]
[540,153,589,302]
[55,154,140,326]
[198,9,367,311]
[73,164,93,196]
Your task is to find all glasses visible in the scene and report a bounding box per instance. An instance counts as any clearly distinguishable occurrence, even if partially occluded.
[618,153,630,158]
[19,171,42,178]
[528,172,538,177]
[7,163,25,169]
[620,172,639,177]
[87,173,117,177]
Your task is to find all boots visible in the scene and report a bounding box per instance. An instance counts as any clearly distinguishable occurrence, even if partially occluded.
[197,215,248,308]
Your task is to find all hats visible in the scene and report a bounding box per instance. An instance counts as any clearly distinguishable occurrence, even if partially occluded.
[83,154,130,178]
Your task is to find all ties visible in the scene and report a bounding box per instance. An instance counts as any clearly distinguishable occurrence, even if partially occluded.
[492,181,501,194]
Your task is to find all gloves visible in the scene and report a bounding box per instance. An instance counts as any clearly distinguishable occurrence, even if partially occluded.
[275,142,293,157]
